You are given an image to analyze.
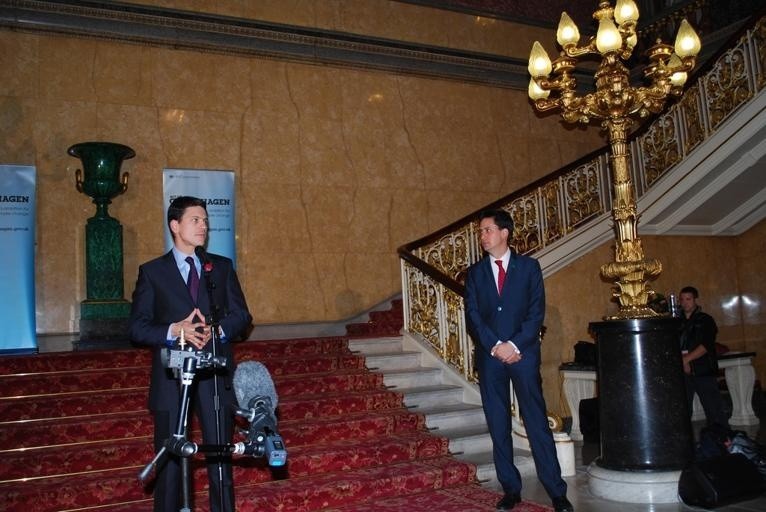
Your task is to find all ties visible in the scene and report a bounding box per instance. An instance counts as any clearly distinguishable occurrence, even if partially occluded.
[185,256,200,304]
[495,260,506,295]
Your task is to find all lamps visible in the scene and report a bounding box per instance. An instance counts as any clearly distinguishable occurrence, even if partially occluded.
[526,0,702,321]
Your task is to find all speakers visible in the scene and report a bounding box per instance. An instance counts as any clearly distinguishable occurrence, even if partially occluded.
[678,452,766,509]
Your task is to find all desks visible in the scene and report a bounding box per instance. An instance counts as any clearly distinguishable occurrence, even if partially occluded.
[558,352,762,441]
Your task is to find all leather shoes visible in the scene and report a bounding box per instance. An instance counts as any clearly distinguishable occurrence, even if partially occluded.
[496,491,522,510]
[551,497,574,511]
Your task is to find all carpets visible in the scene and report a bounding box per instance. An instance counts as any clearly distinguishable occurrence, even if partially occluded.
[2,349,557,512]
[345,298,407,338]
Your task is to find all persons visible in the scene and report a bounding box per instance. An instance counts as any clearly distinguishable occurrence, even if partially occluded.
[700,422,766,472]
[464,211,575,512]
[669,287,731,434]
[126,196,254,512]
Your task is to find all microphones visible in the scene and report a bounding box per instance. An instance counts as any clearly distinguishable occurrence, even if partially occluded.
[194,245,213,273]
[229,360,287,467]
